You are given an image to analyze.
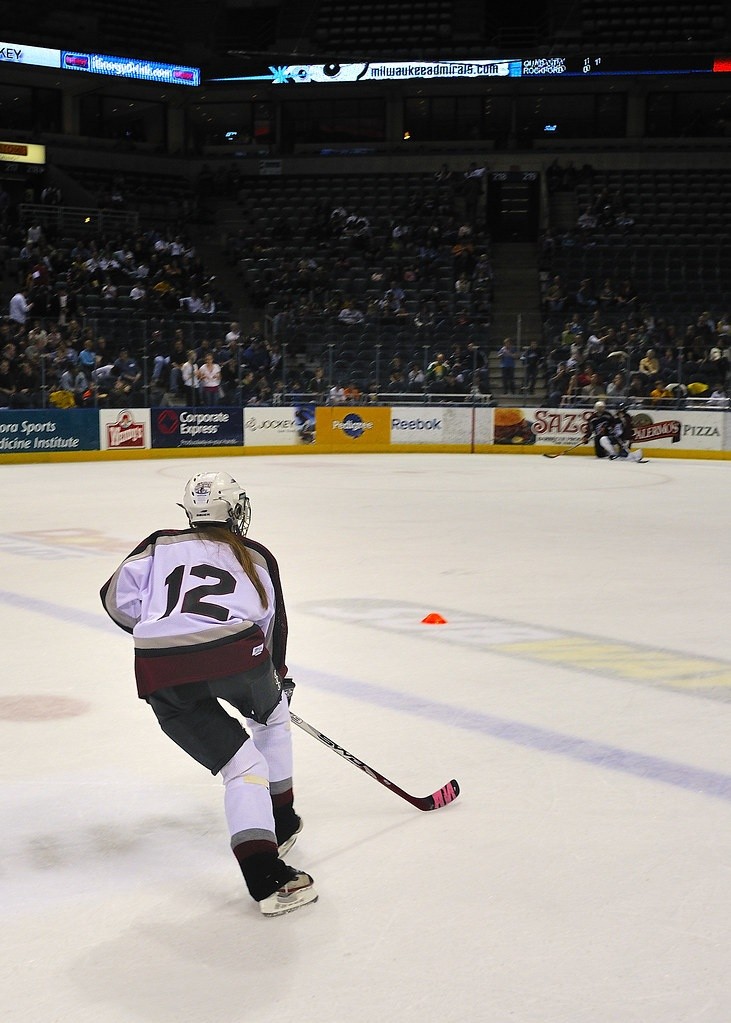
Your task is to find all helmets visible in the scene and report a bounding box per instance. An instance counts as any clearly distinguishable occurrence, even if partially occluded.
[595,402,607,413]
[184,472,251,538]
[616,403,628,411]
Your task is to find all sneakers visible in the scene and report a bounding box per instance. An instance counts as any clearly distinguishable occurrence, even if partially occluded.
[259,869,318,917]
[610,454,620,460]
[277,819,303,858]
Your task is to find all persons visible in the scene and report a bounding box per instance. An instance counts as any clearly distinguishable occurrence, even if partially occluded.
[583,401,635,461]
[0,160,731,412]
[100,471,318,917]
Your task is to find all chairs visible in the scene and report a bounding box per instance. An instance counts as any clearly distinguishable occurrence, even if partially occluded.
[0,0,731,411]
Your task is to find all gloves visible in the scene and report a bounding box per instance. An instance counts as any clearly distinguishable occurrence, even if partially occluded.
[282,678,296,707]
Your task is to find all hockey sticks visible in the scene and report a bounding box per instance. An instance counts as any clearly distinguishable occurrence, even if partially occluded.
[614,435,649,464]
[543,435,594,458]
[290,711,460,811]
